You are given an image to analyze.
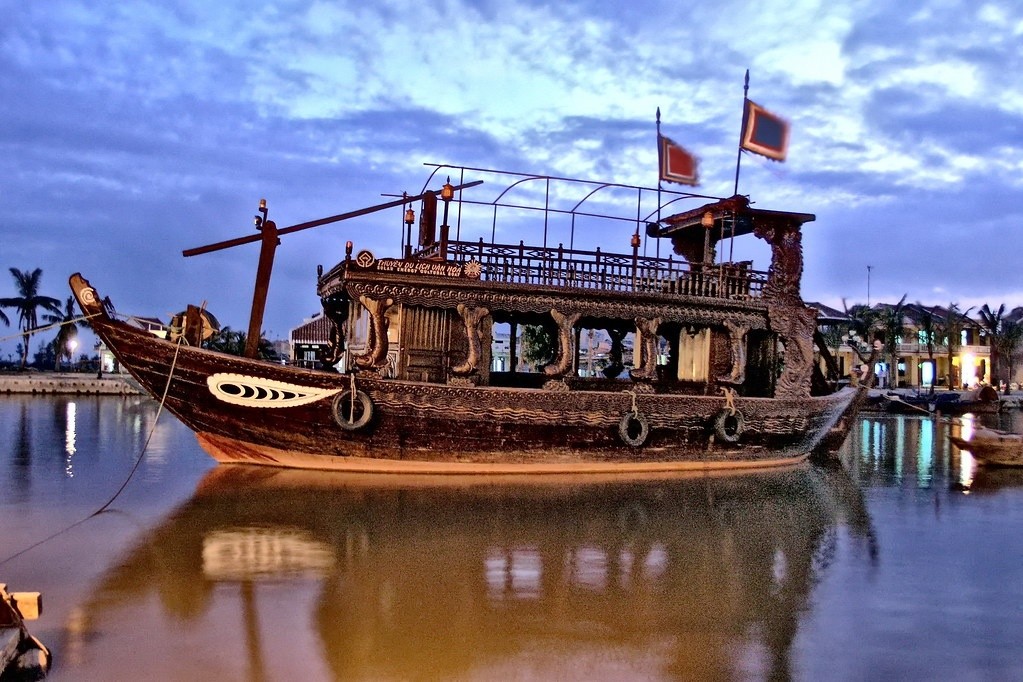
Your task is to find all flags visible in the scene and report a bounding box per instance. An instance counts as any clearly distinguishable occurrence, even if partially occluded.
[740,99,791,162]
[657,135,700,186]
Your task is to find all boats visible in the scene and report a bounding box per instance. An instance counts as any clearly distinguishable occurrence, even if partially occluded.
[948,423,1020,464]
[67,158,868,474]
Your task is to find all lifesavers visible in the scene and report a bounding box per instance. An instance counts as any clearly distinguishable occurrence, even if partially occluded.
[713,408,744,442]
[618,412,647,448]
[331,389,376,434]
